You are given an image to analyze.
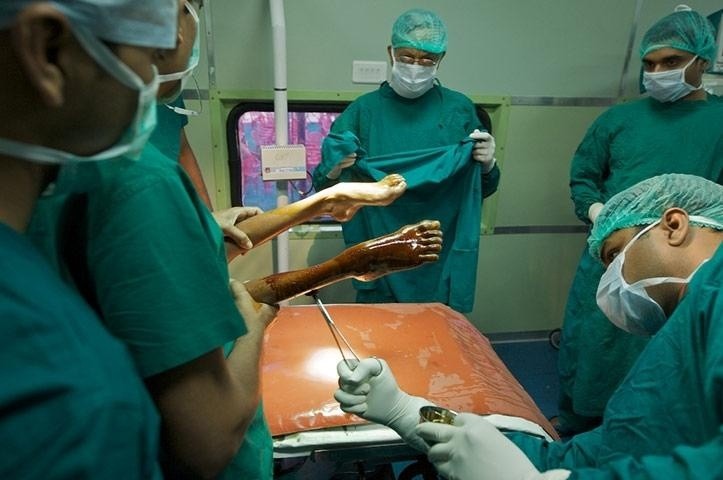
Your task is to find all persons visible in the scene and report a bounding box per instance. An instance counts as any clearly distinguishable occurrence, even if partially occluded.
[21,0,281,479]
[147,89,216,214]
[539,6,722,436]
[1,0,184,478]
[218,171,443,306]
[333,168,721,480]
[308,9,500,314]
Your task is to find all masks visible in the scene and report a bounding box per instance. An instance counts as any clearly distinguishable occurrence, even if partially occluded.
[162,3,202,102]
[595,222,668,337]
[389,49,440,98]
[644,56,701,102]
[57,23,160,192]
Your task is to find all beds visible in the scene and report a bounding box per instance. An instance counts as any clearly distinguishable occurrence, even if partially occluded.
[259,299,561,480]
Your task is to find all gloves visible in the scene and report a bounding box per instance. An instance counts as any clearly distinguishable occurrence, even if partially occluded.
[333,358,438,445]
[325,153,355,181]
[416,413,570,480]
[470,129,498,174]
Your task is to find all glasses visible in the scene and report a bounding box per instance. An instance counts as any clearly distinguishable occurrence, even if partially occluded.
[398,56,437,65]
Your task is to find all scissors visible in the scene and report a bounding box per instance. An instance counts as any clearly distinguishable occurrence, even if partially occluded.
[313,294,383,396]
